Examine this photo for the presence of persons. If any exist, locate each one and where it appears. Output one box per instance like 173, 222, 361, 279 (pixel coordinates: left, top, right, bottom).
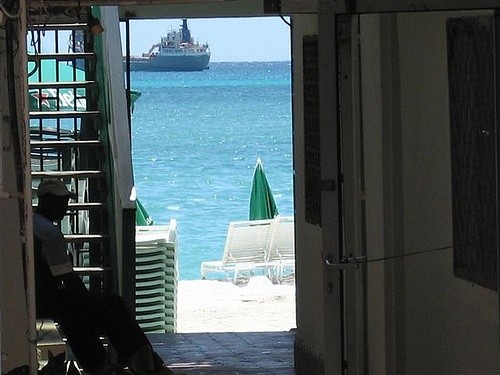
32, 179, 173, 375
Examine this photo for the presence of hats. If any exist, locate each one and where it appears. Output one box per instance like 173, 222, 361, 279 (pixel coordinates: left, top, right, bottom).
36, 177, 77, 202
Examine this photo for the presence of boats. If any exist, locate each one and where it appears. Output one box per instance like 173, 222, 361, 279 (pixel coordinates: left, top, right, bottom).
66, 18, 212, 74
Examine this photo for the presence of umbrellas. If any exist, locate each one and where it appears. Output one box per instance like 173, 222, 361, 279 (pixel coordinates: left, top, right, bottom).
135, 199, 153, 226
28, 51, 142, 115
249, 157, 279, 226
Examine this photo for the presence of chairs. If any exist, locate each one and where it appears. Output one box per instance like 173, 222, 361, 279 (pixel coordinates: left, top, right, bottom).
201, 216, 295, 286
